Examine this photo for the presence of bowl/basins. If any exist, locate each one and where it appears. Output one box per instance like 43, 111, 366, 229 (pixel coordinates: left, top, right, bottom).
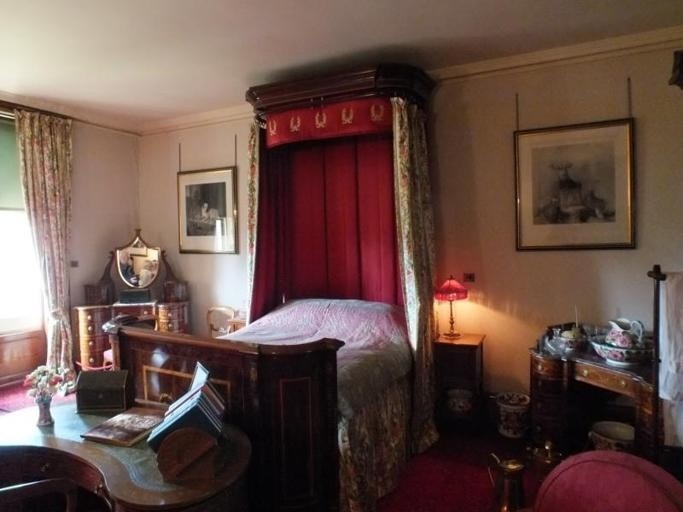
554, 334, 586, 350
589, 336, 653, 365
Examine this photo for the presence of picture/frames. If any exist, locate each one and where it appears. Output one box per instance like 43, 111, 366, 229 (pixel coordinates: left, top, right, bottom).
512, 118, 637, 252
175, 164, 238, 254
129, 238, 147, 257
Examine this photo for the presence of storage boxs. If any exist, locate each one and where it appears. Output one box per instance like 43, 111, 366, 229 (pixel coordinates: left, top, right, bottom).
78, 371, 135, 413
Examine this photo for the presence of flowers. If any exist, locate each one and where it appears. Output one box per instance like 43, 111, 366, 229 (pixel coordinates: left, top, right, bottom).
22, 365, 62, 403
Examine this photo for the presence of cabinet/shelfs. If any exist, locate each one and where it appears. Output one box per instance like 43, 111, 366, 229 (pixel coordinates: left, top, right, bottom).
70, 298, 191, 372
529, 347, 674, 474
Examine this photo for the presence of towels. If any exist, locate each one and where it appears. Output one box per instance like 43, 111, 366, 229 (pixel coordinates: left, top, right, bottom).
658, 271, 682, 446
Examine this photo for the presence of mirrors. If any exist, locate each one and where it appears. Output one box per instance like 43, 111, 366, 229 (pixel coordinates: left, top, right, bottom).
113, 228, 162, 289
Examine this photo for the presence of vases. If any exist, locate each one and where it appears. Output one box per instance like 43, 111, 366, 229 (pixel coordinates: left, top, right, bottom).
34, 397, 54, 428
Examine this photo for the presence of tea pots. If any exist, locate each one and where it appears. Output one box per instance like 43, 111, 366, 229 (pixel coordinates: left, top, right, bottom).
606, 319, 645, 349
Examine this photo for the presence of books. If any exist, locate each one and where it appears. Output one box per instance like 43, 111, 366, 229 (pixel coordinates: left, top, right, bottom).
147, 382, 227, 452
79, 407, 166, 448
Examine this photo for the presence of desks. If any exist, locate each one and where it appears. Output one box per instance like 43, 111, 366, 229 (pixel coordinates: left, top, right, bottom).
0, 397, 251, 511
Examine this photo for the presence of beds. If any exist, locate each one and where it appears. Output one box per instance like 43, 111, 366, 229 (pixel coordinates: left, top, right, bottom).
101, 296, 434, 512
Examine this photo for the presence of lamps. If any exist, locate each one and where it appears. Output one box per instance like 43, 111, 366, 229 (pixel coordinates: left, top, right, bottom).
434, 275, 466, 340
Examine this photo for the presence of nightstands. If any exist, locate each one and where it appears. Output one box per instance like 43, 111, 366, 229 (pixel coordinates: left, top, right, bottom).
227, 317, 246, 334
432, 332, 485, 421
